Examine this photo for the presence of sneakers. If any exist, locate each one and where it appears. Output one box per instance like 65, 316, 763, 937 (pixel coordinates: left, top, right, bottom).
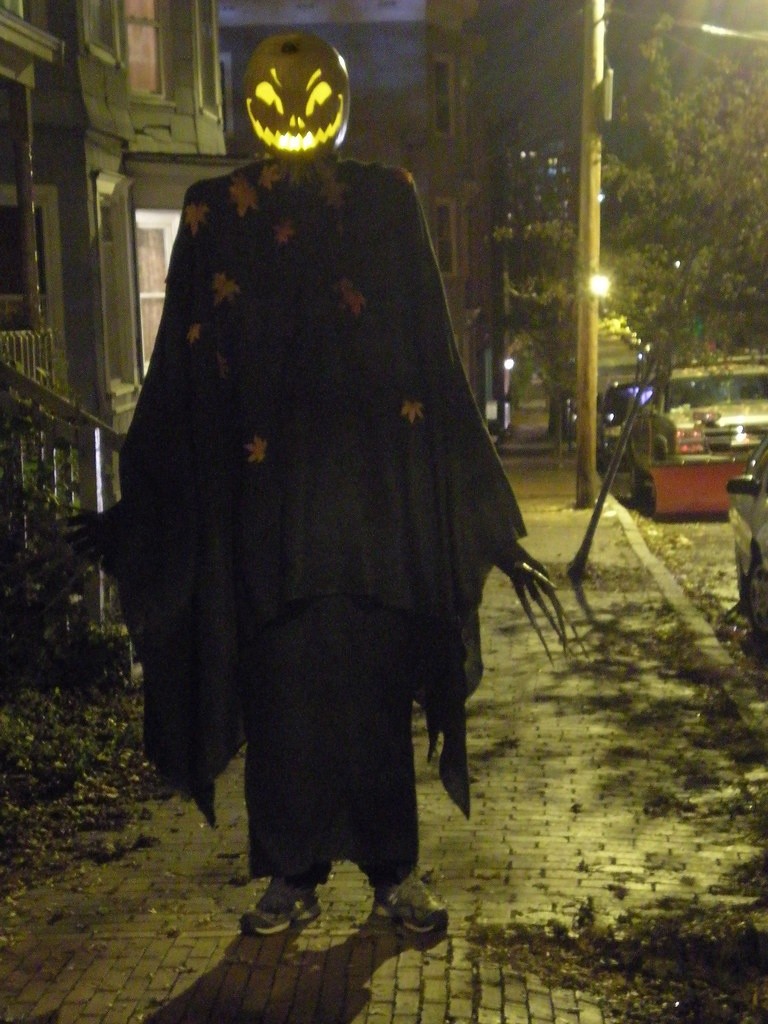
238, 880, 322, 935
373, 864, 448, 933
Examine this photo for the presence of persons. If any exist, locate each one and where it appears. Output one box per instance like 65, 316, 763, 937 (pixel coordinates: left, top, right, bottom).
11, 31, 590, 935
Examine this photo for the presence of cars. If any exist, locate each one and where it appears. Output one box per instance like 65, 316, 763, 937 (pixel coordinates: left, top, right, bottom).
725, 431, 768, 635
603, 375, 642, 470
630, 363, 768, 502
